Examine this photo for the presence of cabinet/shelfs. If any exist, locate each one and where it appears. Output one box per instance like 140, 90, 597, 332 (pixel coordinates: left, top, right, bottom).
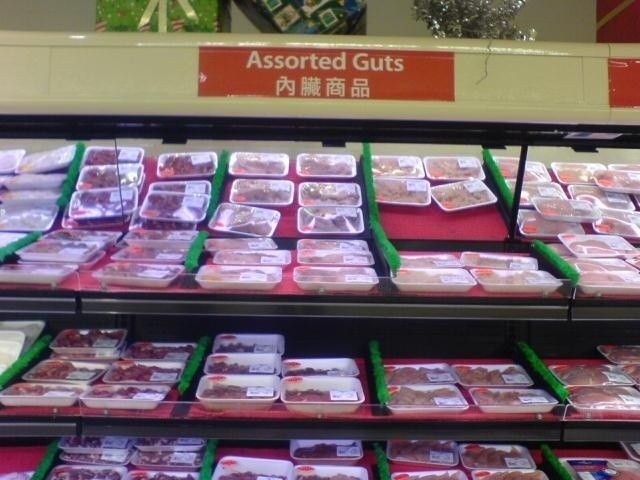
1, 113, 639, 479
